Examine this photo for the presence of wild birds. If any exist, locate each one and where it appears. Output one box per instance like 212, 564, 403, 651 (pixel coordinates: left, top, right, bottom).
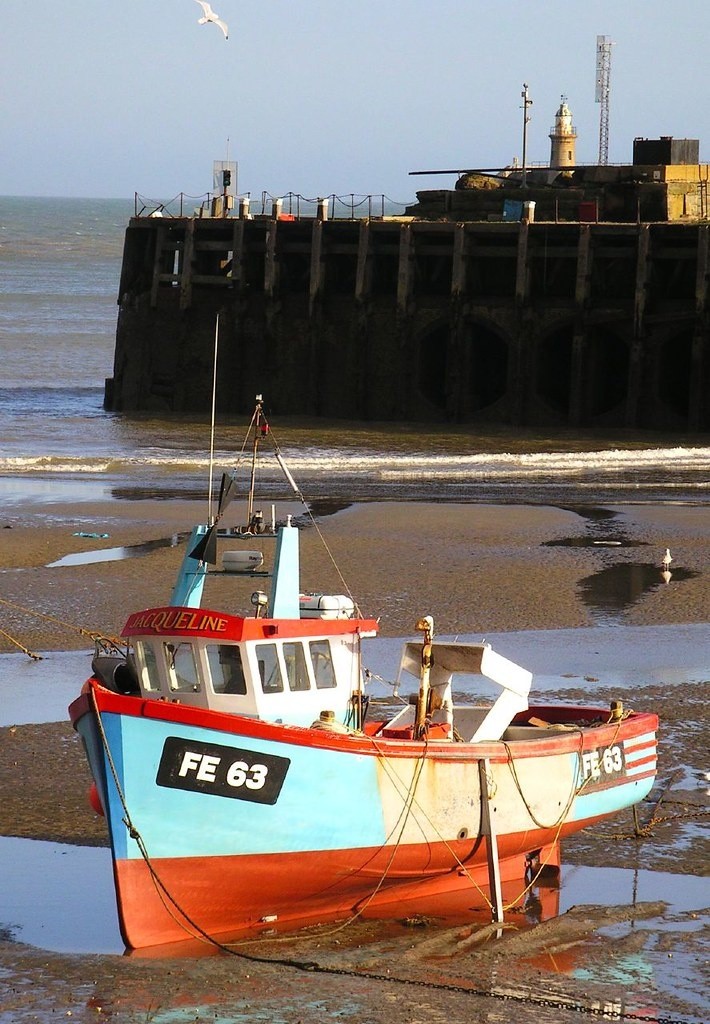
661, 548, 672, 567
660, 567, 672, 585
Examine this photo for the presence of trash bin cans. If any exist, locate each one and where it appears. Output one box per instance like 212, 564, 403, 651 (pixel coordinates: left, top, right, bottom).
523, 201, 537, 223
211, 196, 223, 218
239, 197, 250, 220
272, 198, 284, 221
317, 199, 329, 221
578, 201, 596, 223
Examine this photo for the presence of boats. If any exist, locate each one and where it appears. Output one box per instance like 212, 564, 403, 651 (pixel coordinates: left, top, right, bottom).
69, 394, 658, 959
